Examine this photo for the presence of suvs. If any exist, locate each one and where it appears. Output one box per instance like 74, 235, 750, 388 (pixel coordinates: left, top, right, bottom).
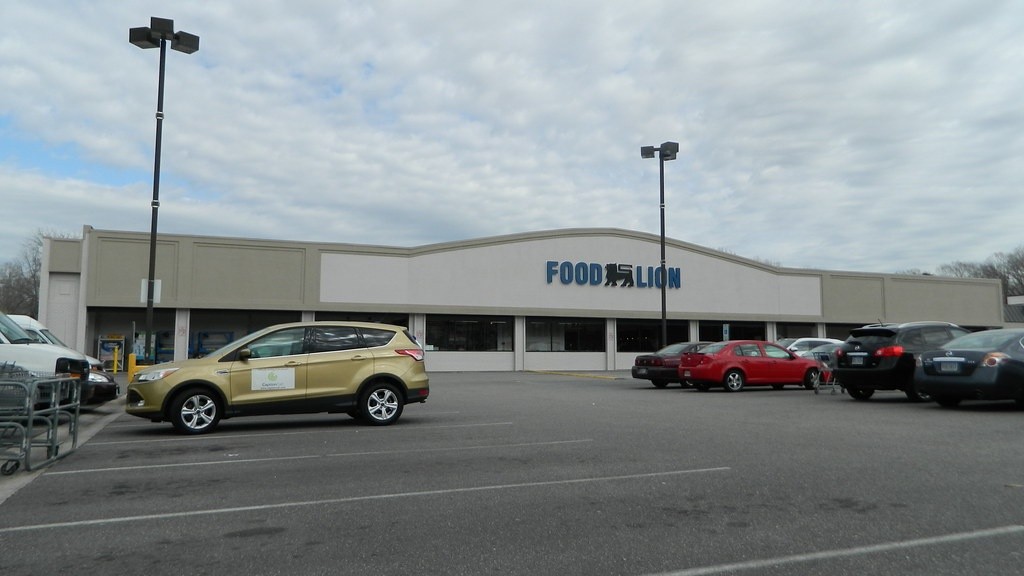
126, 320, 429, 435
830, 320, 974, 403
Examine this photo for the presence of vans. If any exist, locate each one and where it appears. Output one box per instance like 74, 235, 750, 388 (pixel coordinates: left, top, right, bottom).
0, 312, 90, 426
9, 315, 105, 375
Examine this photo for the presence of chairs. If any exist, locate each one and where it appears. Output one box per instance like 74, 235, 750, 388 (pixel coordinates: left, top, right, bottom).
290, 333, 305, 354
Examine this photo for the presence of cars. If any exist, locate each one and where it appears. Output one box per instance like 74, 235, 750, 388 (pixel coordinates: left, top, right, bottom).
752, 337, 845, 358
915, 327, 1024, 409
631, 341, 716, 388
679, 340, 832, 393
81, 368, 120, 411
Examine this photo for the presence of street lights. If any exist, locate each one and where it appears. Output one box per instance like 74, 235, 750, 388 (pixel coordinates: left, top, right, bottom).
641, 140, 680, 352
129, 17, 200, 368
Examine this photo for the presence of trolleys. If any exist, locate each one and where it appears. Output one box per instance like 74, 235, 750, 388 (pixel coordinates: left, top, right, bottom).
0, 364, 72, 476
811, 350, 847, 394
